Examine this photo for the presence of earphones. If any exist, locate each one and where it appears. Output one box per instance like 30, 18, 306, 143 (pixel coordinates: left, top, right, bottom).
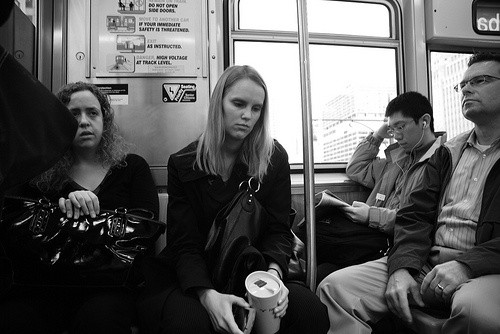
422, 121, 426, 127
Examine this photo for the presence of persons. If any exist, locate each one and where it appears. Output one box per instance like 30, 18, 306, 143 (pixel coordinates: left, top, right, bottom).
314, 46, 500, 334
296, 91, 448, 289
160, 64, 330, 334
24, 81, 158, 334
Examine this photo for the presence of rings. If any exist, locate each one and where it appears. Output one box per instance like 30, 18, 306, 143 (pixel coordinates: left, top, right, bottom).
438, 283, 444, 291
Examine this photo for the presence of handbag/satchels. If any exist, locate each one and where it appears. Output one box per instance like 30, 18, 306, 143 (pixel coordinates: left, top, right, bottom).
314, 209, 391, 266
1, 194, 167, 293
204, 176, 307, 283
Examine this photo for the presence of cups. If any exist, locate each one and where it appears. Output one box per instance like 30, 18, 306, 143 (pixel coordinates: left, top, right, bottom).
244, 271, 282, 334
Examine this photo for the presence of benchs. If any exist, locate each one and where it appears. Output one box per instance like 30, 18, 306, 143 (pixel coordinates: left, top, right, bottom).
409, 304, 450, 334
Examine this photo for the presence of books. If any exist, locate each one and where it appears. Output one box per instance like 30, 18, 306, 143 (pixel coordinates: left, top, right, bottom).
300, 188, 352, 228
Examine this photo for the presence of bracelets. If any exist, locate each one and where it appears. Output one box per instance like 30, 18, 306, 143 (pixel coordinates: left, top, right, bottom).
268, 268, 282, 280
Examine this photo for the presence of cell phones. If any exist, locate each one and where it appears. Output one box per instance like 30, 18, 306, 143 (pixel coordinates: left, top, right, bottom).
235, 305, 256, 334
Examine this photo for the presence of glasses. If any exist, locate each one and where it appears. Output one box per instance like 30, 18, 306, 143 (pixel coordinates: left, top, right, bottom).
453, 74, 500, 93
386, 119, 415, 138
